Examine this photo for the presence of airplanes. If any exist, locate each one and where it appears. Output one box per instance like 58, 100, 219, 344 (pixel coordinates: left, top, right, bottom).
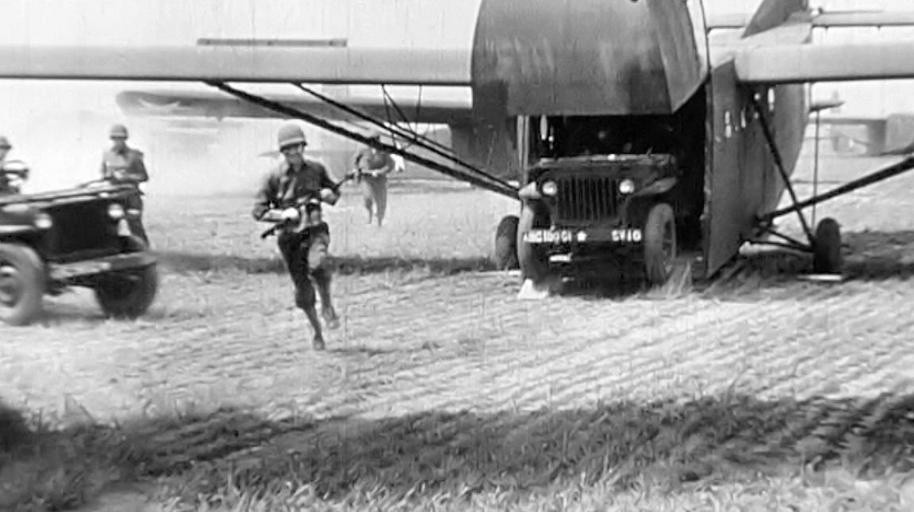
0, 0, 914, 288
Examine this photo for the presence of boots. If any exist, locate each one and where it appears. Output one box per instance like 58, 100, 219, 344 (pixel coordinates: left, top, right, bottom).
320, 293, 340, 329
307, 313, 325, 352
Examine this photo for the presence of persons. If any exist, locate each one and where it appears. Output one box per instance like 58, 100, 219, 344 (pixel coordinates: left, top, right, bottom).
353, 132, 394, 226
100, 125, 149, 247
0, 138, 28, 198
252, 125, 339, 351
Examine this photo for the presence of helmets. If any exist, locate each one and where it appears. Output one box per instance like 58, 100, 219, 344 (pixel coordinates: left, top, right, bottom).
0, 136, 11, 148
367, 129, 379, 139
277, 124, 306, 152
109, 123, 128, 140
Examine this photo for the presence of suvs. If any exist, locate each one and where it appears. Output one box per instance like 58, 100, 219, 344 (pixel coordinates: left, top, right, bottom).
513, 144, 684, 287
0, 159, 162, 327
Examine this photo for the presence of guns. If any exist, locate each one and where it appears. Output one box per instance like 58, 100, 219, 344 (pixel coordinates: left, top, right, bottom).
261, 173, 356, 238
